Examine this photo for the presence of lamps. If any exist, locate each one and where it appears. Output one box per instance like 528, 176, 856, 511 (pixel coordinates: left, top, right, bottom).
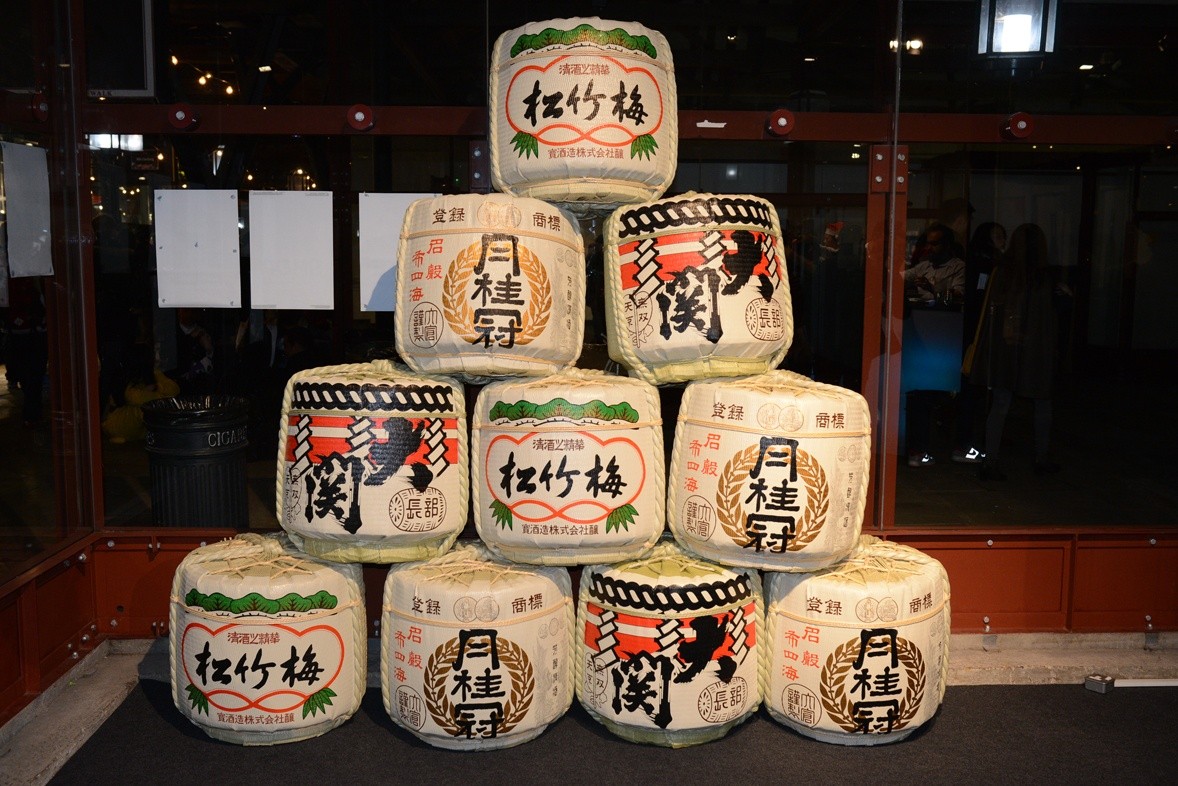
968, 0, 1063, 120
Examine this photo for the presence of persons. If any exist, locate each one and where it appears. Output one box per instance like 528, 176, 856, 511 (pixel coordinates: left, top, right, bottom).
0, 115, 1013, 503
974, 223, 1063, 482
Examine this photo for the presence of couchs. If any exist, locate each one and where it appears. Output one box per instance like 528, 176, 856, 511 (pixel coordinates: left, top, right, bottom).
841, 299, 964, 396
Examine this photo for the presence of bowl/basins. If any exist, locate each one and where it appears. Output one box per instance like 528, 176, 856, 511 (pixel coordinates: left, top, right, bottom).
923, 299, 936, 307
